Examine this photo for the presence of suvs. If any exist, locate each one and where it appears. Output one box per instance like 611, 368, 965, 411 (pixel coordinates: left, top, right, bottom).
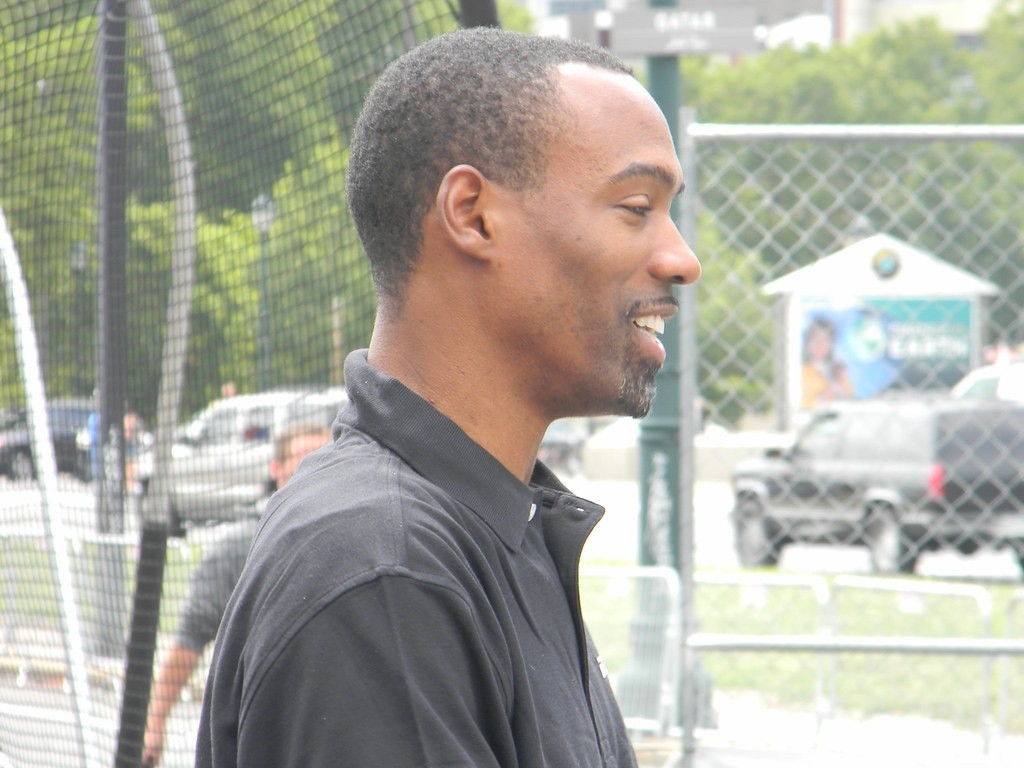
727, 391, 1024, 574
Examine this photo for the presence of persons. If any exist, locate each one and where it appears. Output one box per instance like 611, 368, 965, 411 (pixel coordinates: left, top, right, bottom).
86, 389, 145, 517
801, 319, 854, 409
140, 421, 334, 767
194, 26, 699, 768
981, 339, 1024, 367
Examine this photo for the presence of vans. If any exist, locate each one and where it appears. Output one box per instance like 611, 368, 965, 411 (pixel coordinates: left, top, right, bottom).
135, 393, 345, 535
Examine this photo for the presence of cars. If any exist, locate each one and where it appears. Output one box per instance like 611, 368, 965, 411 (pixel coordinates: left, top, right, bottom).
0, 400, 105, 481
